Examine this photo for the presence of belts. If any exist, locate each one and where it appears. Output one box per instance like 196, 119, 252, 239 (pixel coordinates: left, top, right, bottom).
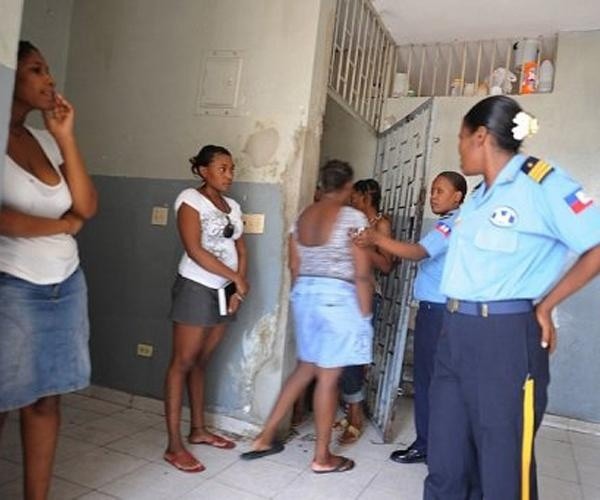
445, 299, 532, 317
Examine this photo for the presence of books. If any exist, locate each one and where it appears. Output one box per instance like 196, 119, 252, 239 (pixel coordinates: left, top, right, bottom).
218, 282, 237, 316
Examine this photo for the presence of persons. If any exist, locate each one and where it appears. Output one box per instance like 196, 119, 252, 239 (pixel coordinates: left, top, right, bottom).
160, 144, 252, 474
294, 184, 324, 429
346, 169, 468, 465
0, 39, 101, 500
421, 95, 600, 499
238, 157, 377, 474
332, 179, 396, 446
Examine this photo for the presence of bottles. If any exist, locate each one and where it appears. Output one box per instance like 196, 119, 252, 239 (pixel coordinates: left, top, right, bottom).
450, 78, 502, 95
540, 59, 554, 94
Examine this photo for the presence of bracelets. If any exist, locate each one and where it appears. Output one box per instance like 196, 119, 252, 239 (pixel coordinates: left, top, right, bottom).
234, 292, 244, 301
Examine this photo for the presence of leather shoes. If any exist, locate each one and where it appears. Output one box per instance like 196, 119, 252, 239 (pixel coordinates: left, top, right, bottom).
390, 446, 427, 464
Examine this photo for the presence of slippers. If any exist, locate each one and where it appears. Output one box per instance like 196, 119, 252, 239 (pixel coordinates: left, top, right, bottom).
190, 433, 236, 449
242, 444, 284, 459
164, 451, 206, 472
314, 455, 355, 473
332, 417, 350, 431
335, 423, 363, 447
